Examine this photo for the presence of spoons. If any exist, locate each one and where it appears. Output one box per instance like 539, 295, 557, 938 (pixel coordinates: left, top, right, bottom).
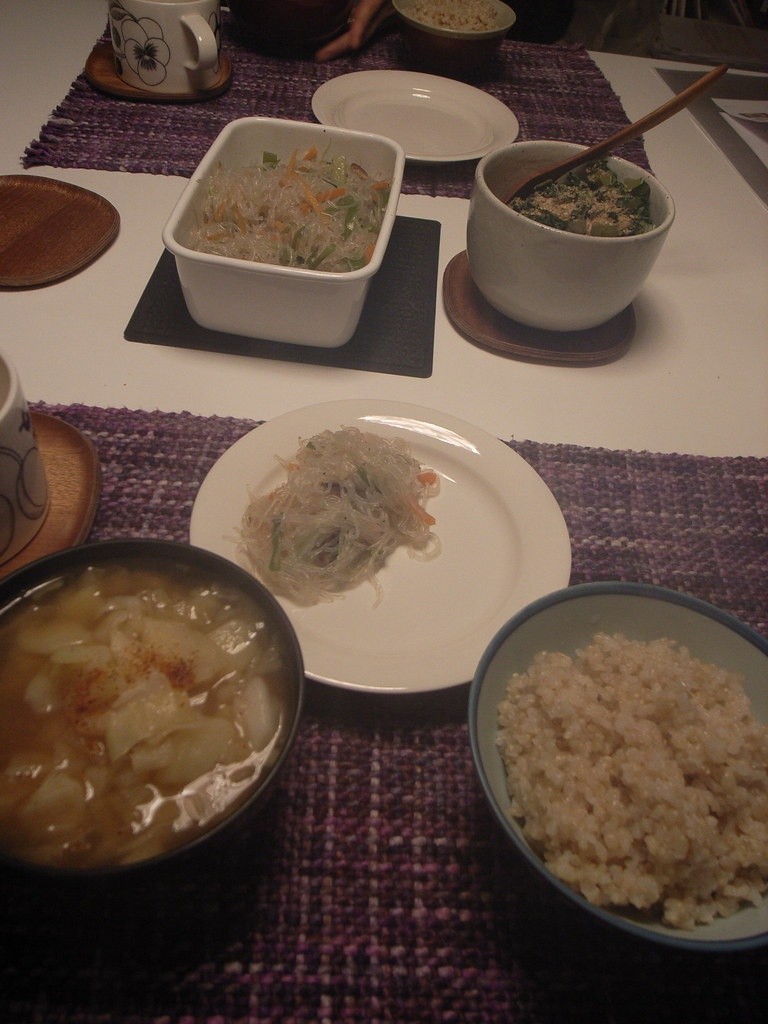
501, 64, 729, 205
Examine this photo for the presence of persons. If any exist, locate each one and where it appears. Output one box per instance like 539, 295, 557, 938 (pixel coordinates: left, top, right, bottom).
315, 0, 577, 63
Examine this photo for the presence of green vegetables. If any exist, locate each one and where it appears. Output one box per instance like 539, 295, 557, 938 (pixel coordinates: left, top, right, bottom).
235, 157, 390, 271
511, 159, 653, 238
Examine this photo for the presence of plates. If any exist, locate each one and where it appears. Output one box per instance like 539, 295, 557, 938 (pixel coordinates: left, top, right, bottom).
311, 70, 520, 165
189, 398, 571, 694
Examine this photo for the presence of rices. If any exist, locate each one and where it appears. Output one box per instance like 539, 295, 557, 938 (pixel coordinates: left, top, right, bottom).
400, 0, 498, 31
492, 632, 768, 930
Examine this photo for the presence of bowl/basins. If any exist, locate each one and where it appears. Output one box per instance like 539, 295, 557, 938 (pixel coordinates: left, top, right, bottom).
466, 140, 675, 331
0, 538, 305, 879
391, 0, 517, 72
469, 581, 768, 950
161, 116, 405, 349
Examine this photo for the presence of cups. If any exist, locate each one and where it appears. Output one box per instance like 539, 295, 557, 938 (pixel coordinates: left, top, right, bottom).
108, 0, 222, 94
0, 357, 50, 565
